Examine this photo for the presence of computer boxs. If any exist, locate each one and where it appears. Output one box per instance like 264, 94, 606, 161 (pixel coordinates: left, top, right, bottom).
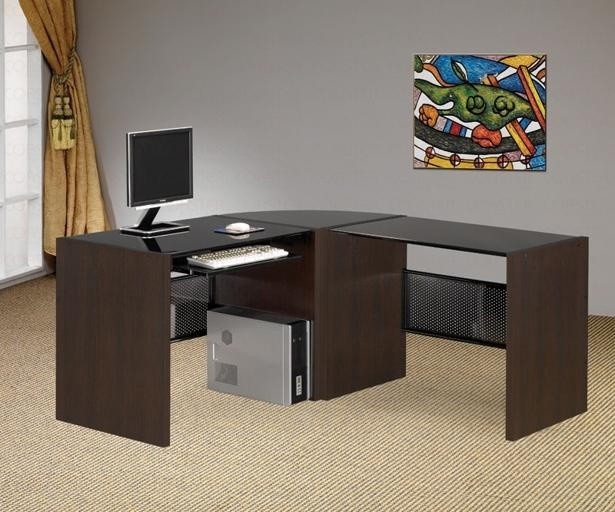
206, 309, 313, 406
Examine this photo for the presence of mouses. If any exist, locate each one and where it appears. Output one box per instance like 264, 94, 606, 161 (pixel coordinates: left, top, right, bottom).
225, 221, 251, 233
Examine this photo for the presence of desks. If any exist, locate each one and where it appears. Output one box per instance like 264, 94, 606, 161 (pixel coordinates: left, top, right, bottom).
54, 210, 590, 448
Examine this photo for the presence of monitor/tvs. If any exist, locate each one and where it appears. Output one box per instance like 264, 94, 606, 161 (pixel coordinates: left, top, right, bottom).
119, 126, 194, 237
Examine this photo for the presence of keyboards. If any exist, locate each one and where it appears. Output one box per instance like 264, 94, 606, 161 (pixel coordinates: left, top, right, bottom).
187, 243, 289, 271
